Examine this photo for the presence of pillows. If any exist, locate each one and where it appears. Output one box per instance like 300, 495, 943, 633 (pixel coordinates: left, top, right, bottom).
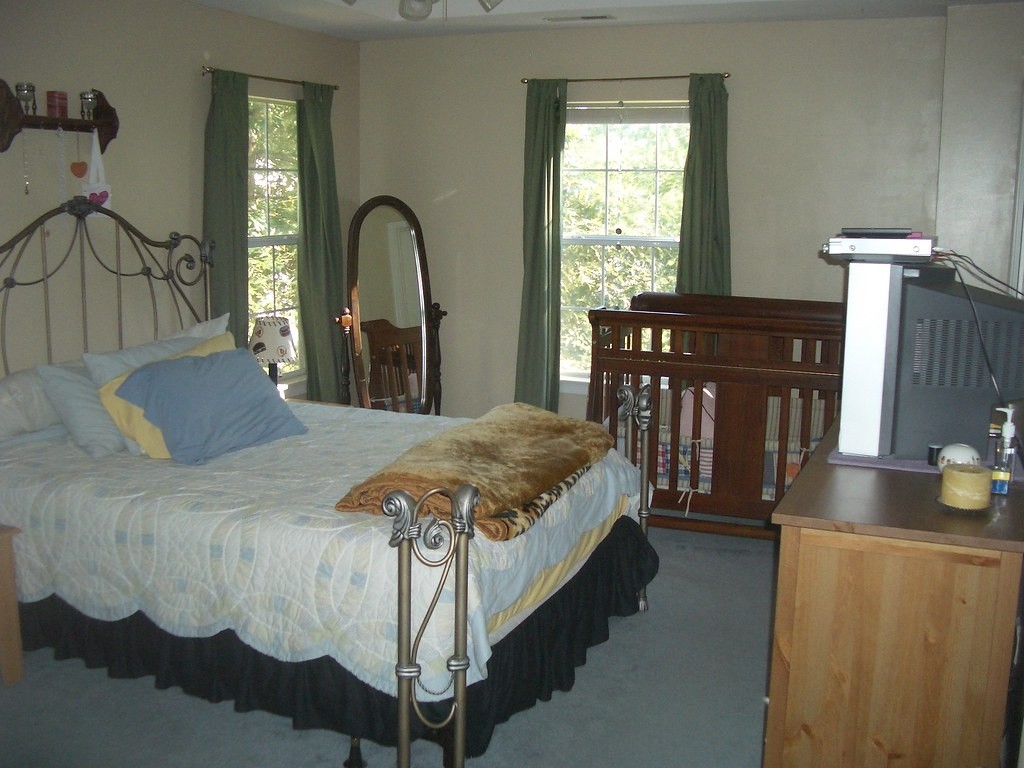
38, 311, 308, 468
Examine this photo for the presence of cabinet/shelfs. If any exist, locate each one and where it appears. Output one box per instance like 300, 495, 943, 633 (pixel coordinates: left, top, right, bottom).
761, 404, 1024, 768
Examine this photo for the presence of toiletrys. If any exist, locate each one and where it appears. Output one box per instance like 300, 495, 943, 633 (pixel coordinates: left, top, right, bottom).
991, 407, 1016, 495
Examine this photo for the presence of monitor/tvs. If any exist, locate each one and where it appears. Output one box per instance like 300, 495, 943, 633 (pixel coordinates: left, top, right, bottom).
838, 262, 1024, 463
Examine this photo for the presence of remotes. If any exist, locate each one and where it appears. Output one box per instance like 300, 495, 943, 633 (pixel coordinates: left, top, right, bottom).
842, 227, 912, 239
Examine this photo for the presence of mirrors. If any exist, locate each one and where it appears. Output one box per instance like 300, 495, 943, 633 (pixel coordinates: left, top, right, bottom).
330, 195, 447, 416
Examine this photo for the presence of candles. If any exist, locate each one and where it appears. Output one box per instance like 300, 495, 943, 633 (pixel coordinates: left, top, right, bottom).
47, 90, 68, 118
941, 457, 992, 510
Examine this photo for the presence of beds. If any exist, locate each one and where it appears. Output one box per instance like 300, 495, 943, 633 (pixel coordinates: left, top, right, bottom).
0, 194, 659, 768
585, 290, 847, 544
360, 320, 422, 415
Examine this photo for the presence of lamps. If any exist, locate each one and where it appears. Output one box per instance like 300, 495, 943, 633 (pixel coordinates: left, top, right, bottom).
0, 77, 119, 196
247, 316, 298, 388
341, 0, 504, 24
